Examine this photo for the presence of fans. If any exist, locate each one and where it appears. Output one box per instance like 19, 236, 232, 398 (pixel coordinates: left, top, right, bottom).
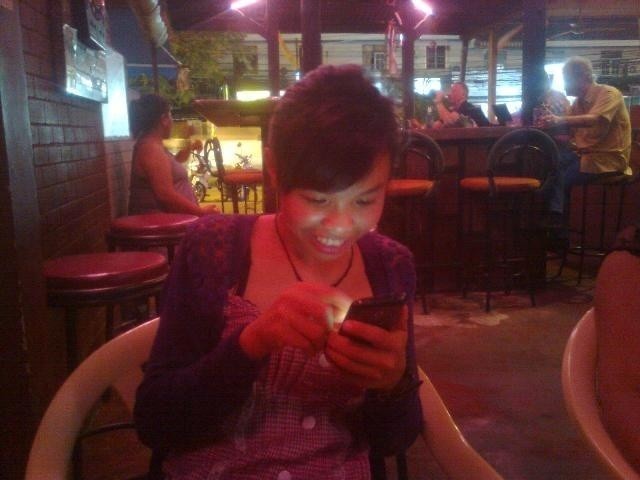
545, 9, 626, 41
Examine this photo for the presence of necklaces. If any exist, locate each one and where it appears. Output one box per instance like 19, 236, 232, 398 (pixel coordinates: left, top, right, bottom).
273, 210, 356, 289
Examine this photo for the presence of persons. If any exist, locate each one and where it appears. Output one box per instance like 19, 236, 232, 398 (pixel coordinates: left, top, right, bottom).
132, 65, 424, 480
530, 55, 632, 253
502, 70, 573, 128
126, 93, 222, 261
430, 82, 490, 130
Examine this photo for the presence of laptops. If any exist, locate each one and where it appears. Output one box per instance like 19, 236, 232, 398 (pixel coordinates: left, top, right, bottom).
467, 107, 504, 127
493, 104, 513, 126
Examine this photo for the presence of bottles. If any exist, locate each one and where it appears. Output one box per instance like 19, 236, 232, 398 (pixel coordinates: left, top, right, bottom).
223, 77, 229, 101
425, 105, 433, 130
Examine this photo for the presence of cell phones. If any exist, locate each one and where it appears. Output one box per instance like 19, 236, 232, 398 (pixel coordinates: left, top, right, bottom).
338, 294, 402, 341
